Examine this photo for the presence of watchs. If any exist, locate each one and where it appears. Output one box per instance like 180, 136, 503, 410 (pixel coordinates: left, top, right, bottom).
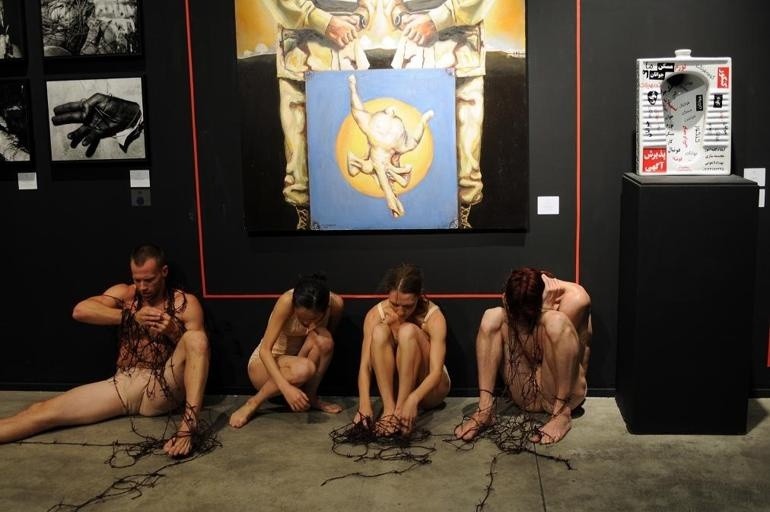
393, 11, 408, 29
353, 11, 367, 29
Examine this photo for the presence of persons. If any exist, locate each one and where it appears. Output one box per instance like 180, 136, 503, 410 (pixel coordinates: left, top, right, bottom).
352, 263, 451, 437
384, 0, 496, 229
454, 265, 593, 445
259, 0, 379, 230
0, 243, 211, 458
229, 269, 341, 429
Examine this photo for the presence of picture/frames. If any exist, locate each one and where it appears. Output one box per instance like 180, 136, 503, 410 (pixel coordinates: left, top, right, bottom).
0, 0, 29, 66
0, 77, 36, 167
43, 74, 148, 164
38, 0, 145, 62
